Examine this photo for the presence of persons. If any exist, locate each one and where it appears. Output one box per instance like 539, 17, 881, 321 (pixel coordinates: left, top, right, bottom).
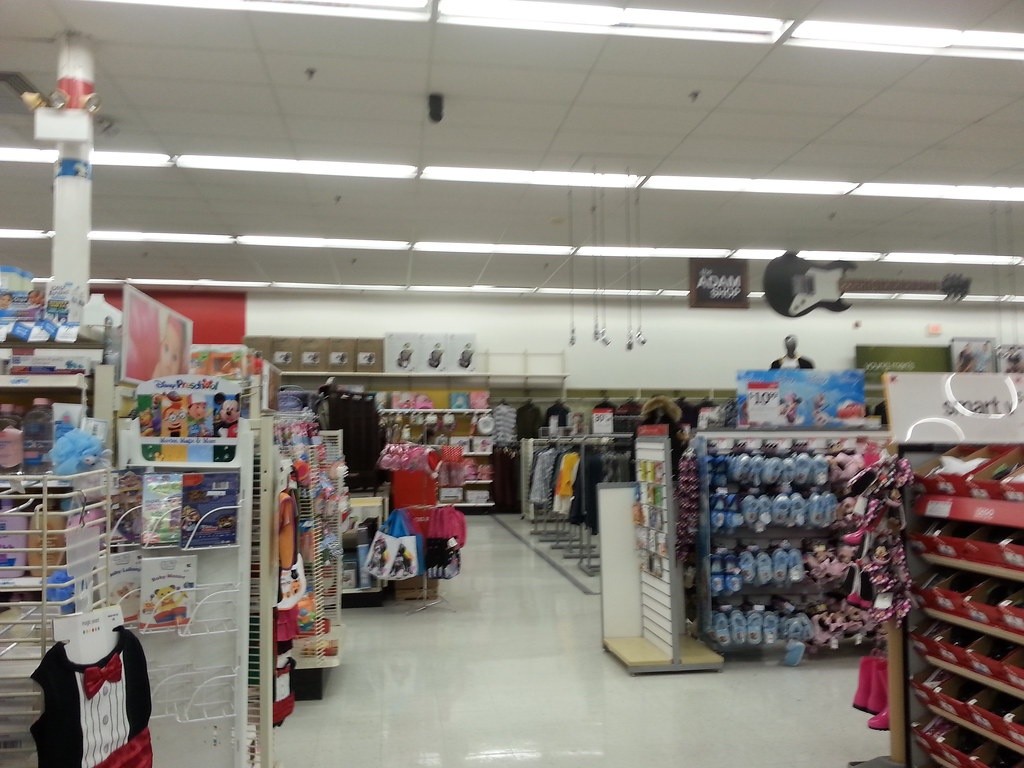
771, 335, 814, 370
153, 314, 186, 380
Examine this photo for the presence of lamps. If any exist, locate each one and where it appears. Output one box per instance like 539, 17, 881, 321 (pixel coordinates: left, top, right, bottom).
19, 87, 119, 143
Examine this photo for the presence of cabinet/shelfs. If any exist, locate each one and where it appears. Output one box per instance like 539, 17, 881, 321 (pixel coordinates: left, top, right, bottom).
517, 368, 1023, 768
0, 282, 493, 768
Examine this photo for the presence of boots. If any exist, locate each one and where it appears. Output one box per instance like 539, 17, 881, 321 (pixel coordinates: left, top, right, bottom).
868, 665, 890, 731
853, 655, 887, 714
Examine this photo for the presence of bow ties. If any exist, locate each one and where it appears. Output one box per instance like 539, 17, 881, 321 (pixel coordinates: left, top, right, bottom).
84, 653, 122, 701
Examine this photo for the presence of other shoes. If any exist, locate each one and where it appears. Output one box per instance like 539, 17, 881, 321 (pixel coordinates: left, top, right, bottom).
847, 593, 872, 610
709, 548, 804, 597
842, 524, 863, 546
784, 642, 805, 666
707, 454, 829, 488
711, 609, 813, 646
710, 492, 838, 531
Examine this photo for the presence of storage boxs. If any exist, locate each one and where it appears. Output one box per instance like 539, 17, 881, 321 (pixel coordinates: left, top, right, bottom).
465, 490, 490, 502
440, 488, 462, 502
